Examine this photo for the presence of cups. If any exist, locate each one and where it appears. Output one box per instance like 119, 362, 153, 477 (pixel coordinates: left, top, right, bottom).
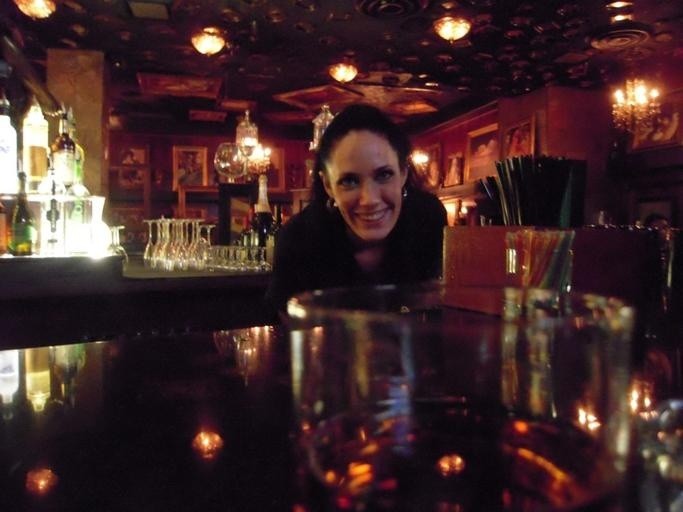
143, 219, 156, 270
204, 223, 216, 273
212, 142, 249, 183
174, 219, 189, 271
256, 246, 274, 272
190, 219, 205, 273
247, 246, 261, 272
217, 245, 226, 273
233, 245, 246, 272
157, 219, 173, 270
227, 245, 236, 272
286, 282, 639, 512
152, 218, 164, 270
105, 225, 129, 272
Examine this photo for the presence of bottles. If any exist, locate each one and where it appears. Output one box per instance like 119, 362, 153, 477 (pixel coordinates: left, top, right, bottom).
636, 221, 641, 225
454, 200, 468, 226
22, 106, 48, 194
8, 172, 37, 256
0, 115, 20, 194
66, 128, 84, 183
236, 204, 258, 247
659, 228, 683, 339
52, 111, 75, 193
251, 175, 273, 248
268, 201, 283, 266
598, 211, 608, 226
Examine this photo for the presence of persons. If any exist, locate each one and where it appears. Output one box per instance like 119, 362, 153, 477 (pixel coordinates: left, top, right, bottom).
272, 103, 448, 311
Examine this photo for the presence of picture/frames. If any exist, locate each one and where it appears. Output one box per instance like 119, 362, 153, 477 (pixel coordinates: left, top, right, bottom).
118, 144, 151, 189
189, 109, 226, 122
466, 122, 498, 179
268, 148, 285, 193
389, 98, 438, 116
498, 111, 537, 161
138, 73, 222, 100
274, 83, 364, 113
219, 183, 254, 246
442, 153, 464, 187
172, 146, 208, 192
217, 97, 256, 115
